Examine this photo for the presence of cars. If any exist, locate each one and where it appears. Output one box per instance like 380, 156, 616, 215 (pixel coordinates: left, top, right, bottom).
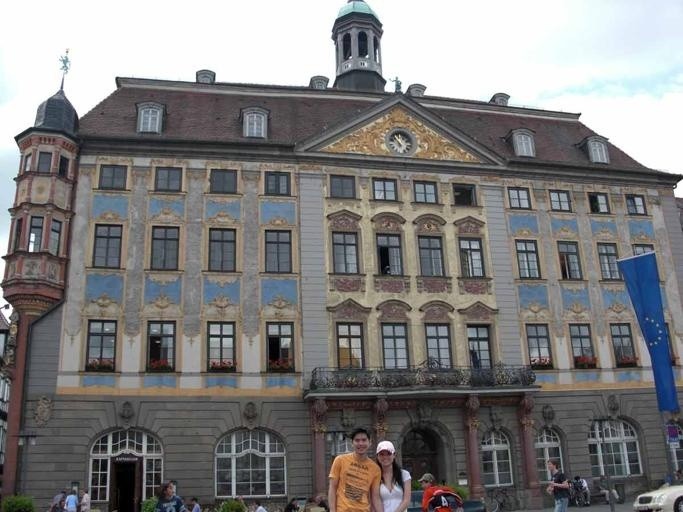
632, 476, 682, 512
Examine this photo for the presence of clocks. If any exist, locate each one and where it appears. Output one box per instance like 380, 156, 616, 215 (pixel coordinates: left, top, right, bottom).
386, 128, 416, 155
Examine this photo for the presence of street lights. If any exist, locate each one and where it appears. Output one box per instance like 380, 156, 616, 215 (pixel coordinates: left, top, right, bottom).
586, 415, 616, 511
11, 430, 43, 498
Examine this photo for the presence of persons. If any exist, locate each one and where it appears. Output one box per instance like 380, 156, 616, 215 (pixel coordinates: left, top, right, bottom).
597, 475, 620, 504
156, 482, 201, 512
50, 490, 91, 512
285, 428, 412, 512
418, 473, 454, 512
547, 459, 591, 512
251, 500, 267, 512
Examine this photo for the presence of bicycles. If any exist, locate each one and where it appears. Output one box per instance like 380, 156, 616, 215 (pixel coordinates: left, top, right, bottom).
567, 480, 586, 507
484, 486, 517, 511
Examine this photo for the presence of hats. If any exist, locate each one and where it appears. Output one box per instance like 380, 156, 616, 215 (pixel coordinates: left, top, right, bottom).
418, 474, 434, 483
377, 441, 395, 455
